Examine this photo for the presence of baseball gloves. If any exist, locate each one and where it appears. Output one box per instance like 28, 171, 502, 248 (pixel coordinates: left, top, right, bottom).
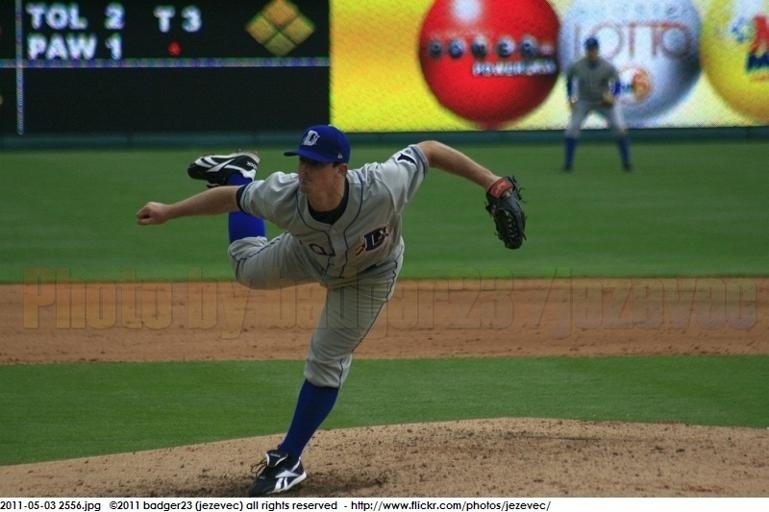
485, 176, 527, 249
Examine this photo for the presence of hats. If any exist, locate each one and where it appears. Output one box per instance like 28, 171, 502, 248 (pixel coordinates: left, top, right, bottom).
583, 41, 599, 51
284, 125, 350, 163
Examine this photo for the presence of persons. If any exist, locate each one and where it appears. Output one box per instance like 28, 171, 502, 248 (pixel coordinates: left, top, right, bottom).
136, 125, 524, 496
563, 37, 632, 172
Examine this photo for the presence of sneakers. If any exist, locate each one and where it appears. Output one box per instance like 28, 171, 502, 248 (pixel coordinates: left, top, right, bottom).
624, 163, 631, 172
188, 152, 260, 187
559, 163, 572, 173
247, 451, 306, 497
